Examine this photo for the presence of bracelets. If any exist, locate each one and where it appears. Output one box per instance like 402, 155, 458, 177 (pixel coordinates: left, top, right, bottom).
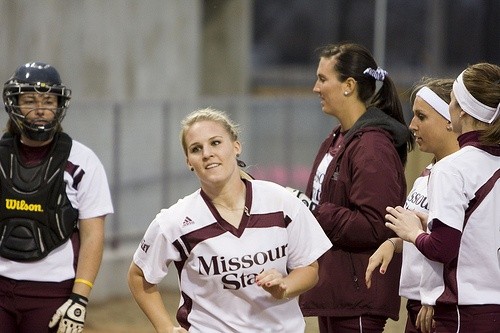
75, 279, 94, 288
388, 238, 397, 249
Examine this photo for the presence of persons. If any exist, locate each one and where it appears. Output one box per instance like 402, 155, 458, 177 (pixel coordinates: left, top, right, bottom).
128, 109, 332, 333
0, 62, 114, 333
366, 79, 461, 333
385, 62, 500, 333
285, 44, 417, 333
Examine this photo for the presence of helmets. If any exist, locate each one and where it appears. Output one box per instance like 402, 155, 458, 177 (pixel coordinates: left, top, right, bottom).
2, 61, 72, 142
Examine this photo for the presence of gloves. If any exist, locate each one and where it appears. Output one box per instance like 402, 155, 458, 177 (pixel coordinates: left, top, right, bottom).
48, 291, 89, 333
285, 186, 318, 212
